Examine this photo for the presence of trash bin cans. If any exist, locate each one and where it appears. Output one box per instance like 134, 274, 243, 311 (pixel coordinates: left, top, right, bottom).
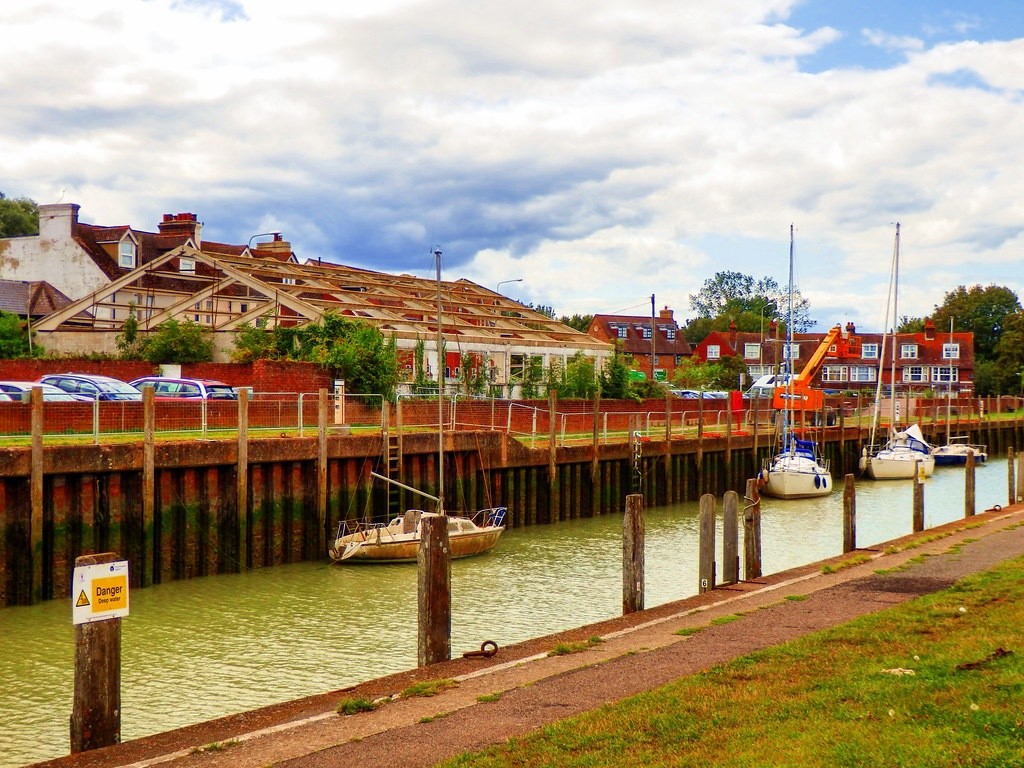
843, 402, 852, 417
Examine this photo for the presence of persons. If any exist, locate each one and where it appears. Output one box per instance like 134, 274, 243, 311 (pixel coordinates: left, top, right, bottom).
846, 322, 856, 336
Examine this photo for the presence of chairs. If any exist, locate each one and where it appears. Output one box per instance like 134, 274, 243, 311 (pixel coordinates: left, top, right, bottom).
160, 386, 169, 392
178, 386, 188, 392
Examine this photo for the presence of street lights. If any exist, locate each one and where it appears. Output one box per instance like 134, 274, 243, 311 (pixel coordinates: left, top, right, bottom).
496, 278, 523, 293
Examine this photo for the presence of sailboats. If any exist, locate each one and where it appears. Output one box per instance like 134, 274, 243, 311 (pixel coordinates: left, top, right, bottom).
931, 315, 988, 467
329, 242, 508, 566
755, 221, 836, 502
857, 221, 937, 481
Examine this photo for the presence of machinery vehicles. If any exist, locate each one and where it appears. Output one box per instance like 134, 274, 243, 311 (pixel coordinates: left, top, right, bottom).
772, 325, 862, 425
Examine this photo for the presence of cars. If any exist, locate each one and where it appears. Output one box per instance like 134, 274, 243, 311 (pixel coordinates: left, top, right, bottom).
672, 389, 772, 399
0, 371, 145, 402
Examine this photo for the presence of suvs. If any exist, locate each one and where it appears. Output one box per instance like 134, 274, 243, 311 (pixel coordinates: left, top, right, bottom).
127, 376, 238, 400
